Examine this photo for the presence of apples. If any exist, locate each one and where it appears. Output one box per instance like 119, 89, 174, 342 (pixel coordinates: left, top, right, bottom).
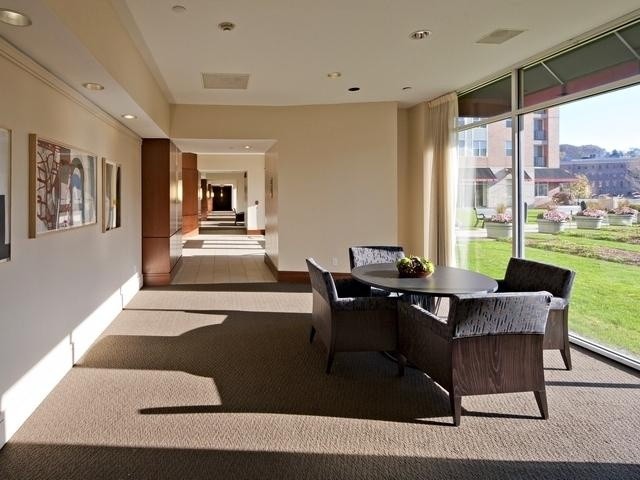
398, 256, 434, 271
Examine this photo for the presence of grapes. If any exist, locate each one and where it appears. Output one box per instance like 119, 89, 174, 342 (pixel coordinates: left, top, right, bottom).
396, 260, 424, 275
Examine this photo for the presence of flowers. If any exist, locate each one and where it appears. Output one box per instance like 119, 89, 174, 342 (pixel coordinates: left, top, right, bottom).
608, 205, 638, 216
576, 208, 606, 218
484, 211, 512, 223
537, 209, 572, 222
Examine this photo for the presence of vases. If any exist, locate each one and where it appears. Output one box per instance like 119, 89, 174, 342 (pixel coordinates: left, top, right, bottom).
607, 215, 634, 227
574, 216, 603, 229
484, 222, 512, 238
536, 219, 562, 233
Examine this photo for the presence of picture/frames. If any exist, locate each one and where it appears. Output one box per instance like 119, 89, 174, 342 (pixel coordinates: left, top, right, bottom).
1, 127, 13, 264
29, 133, 98, 239
102, 157, 122, 233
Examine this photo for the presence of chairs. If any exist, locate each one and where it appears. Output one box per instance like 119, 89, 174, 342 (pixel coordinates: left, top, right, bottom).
232, 207, 245, 225
348, 245, 435, 313
398, 290, 554, 426
305, 258, 397, 373
496, 257, 576, 370
474, 208, 484, 227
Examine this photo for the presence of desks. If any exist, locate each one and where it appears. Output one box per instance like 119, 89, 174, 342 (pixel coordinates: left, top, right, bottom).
351, 262, 499, 371
